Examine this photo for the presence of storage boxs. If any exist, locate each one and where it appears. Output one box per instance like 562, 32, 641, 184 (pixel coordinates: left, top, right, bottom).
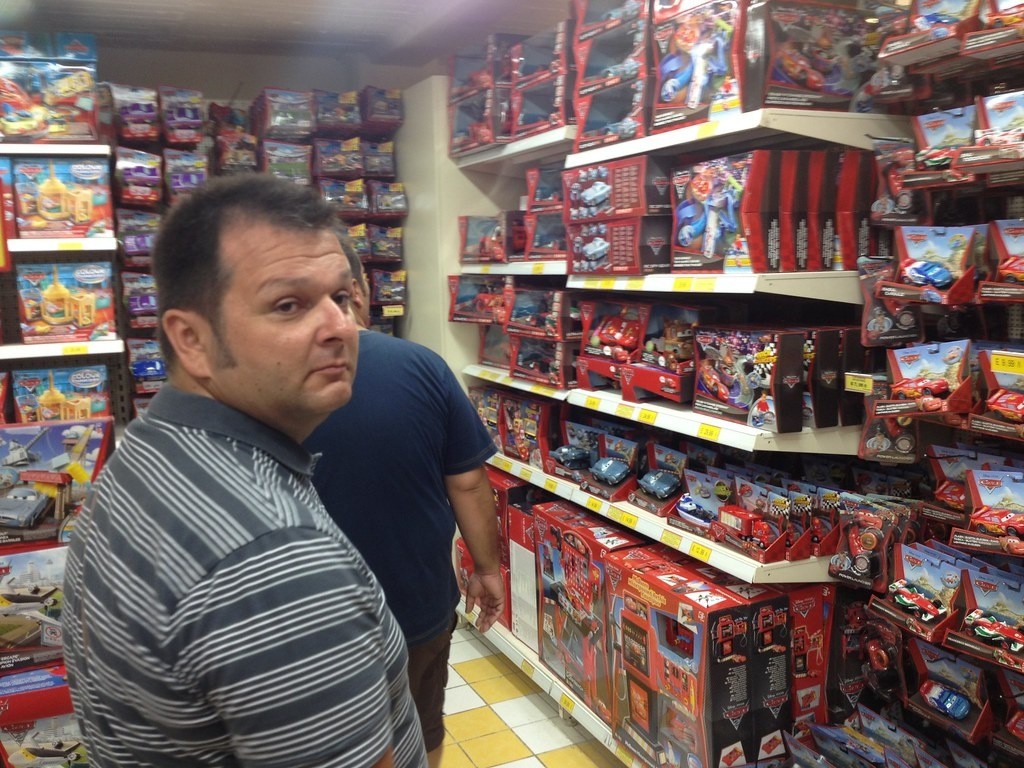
620, 363, 694, 406
453, 463, 837, 768
565, 214, 674, 274
652, 0, 877, 134
690, 325, 804, 434
0, 541, 92, 768
478, 322, 516, 368
502, 285, 585, 339
561, 154, 678, 225
574, 356, 624, 392
450, 0, 650, 156
838, 323, 862, 427
459, 166, 562, 263
0, 32, 410, 539
670, 147, 888, 272
579, 297, 647, 362
448, 276, 518, 325
784, 328, 838, 428
635, 299, 723, 375
507, 330, 579, 389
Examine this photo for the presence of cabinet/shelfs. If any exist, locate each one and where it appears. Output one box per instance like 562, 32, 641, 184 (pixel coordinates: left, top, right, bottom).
0, 63, 129, 428
450, 0, 1024, 768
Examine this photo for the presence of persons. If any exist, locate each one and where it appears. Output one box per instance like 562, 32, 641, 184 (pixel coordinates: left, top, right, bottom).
55, 172, 433, 768
293, 230, 505, 768
